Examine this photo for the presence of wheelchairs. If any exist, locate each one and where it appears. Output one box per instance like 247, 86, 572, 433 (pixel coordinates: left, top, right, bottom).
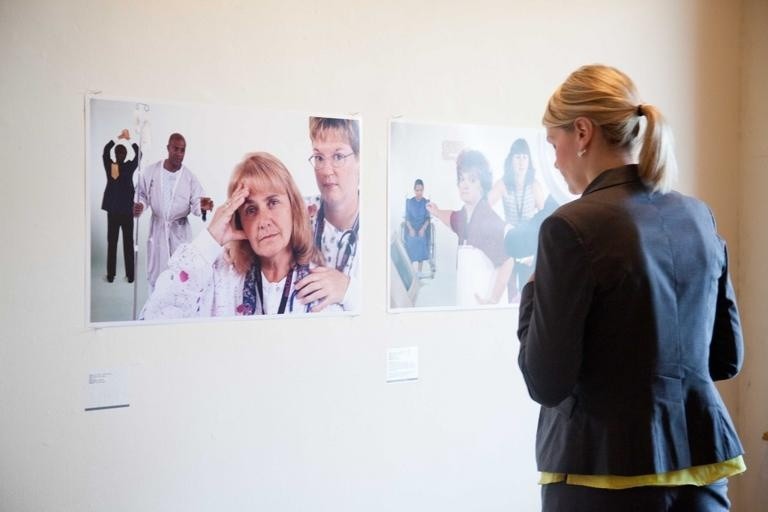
401, 198, 436, 280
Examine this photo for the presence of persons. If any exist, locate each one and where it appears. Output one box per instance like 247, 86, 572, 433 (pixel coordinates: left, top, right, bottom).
101, 128, 143, 283
138, 153, 347, 321
133, 133, 213, 296
516, 64, 748, 512
405, 139, 544, 306
223, 116, 359, 312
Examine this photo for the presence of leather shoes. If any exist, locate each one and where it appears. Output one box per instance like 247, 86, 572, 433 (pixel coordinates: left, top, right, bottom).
108, 275, 114, 282
128, 277, 134, 283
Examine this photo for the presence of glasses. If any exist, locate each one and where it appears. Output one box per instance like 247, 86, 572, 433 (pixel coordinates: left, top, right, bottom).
309, 152, 354, 169
